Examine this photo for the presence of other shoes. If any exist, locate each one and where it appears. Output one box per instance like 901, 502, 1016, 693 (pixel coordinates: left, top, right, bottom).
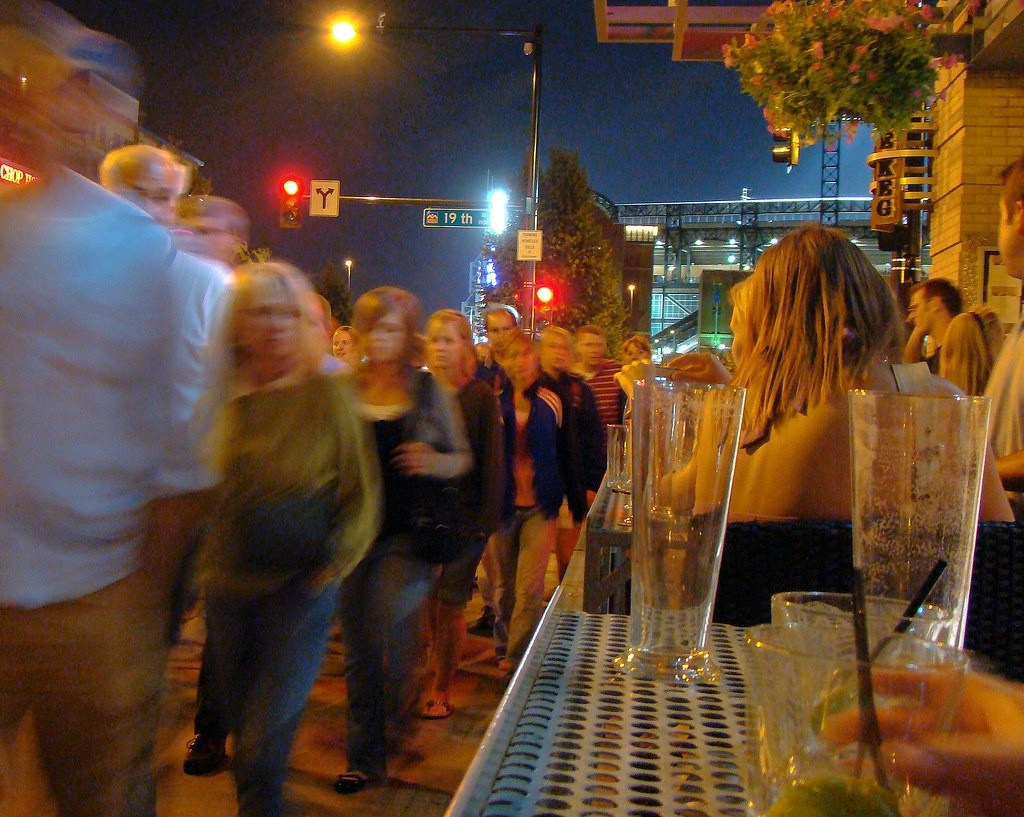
497, 669, 516, 694
335, 769, 387, 794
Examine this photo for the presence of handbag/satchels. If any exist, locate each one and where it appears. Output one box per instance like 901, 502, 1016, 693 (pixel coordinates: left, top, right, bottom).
414, 487, 471, 564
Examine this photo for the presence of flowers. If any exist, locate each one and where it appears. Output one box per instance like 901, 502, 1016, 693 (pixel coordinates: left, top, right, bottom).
721, 0, 959, 139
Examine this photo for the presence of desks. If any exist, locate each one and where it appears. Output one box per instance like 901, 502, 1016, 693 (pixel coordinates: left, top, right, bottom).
584, 464, 635, 617
443, 587, 761, 816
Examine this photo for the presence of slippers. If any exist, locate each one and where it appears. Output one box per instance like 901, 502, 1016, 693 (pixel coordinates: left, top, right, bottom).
422, 698, 454, 717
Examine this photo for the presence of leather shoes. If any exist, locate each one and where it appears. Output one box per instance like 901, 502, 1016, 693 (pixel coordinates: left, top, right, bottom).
467, 607, 495, 636
183, 732, 225, 774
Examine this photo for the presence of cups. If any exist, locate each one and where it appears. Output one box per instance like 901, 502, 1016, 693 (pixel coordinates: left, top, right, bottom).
606, 424, 653, 495
847, 387, 996, 650
744, 623, 969, 817
611, 370, 746, 684
771, 591, 957, 641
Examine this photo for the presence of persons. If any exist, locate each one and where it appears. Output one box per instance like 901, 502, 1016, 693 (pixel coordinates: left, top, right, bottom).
976, 155, 1024, 496
570, 323, 625, 437
901, 278, 964, 373
616, 226, 1013, 521
821, 666, 1024, 817
939, 303, 1005, 396
0, 139, 605, 817
619, 336, 653, 424
